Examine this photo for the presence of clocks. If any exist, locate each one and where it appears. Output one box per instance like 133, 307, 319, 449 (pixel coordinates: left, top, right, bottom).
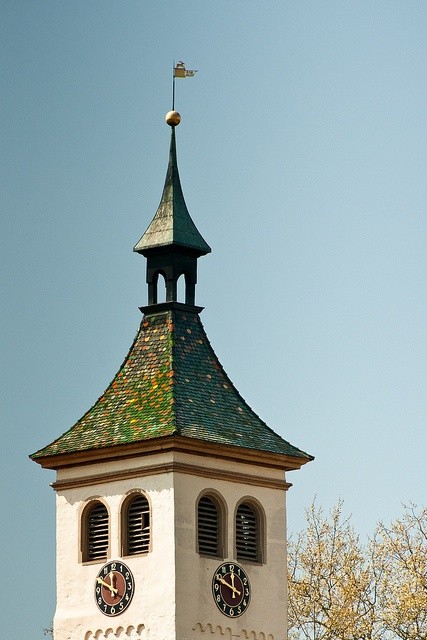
212, 561, 252, 619
93, 560, 134, 617
